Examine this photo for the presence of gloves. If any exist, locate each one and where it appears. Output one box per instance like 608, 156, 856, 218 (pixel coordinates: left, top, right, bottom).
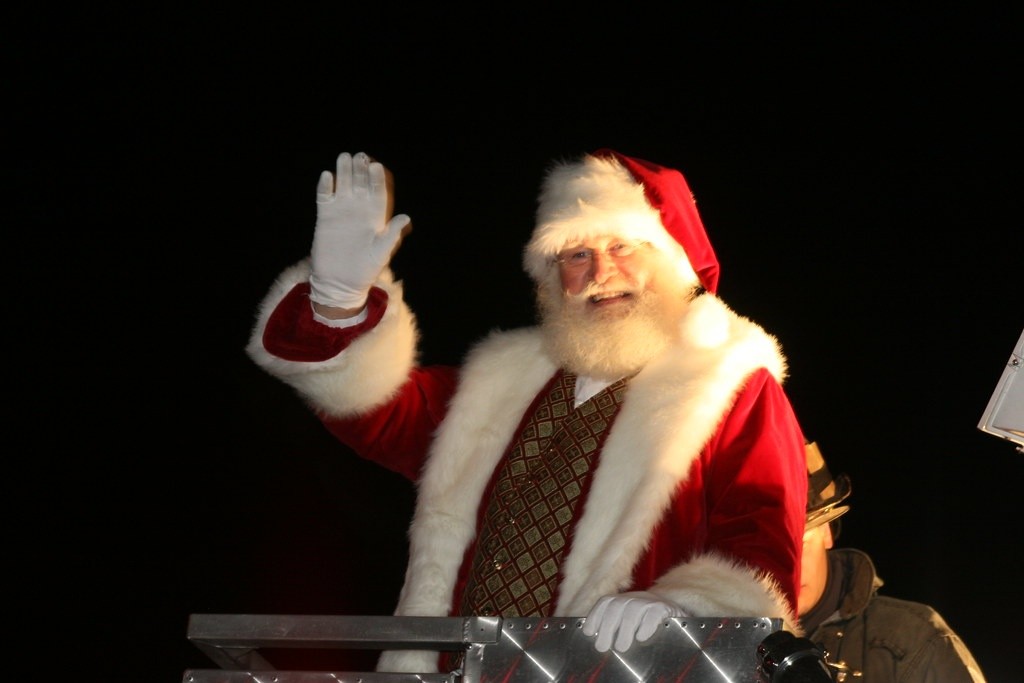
581, 589, 689, 653
308, 152, 412, 311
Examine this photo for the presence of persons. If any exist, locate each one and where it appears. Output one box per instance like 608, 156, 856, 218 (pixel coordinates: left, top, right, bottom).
795, 441, 987, 683
243, 146, 808, 683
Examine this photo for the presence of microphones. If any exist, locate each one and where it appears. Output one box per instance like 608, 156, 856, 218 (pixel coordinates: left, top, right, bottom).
756, 629, 836, 683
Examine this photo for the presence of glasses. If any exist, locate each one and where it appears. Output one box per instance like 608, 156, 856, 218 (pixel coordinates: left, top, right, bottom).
549, 242, 634, 266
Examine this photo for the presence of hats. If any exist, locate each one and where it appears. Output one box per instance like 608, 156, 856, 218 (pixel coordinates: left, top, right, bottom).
520, 148, 721, 296
805, 441, 853, 531
